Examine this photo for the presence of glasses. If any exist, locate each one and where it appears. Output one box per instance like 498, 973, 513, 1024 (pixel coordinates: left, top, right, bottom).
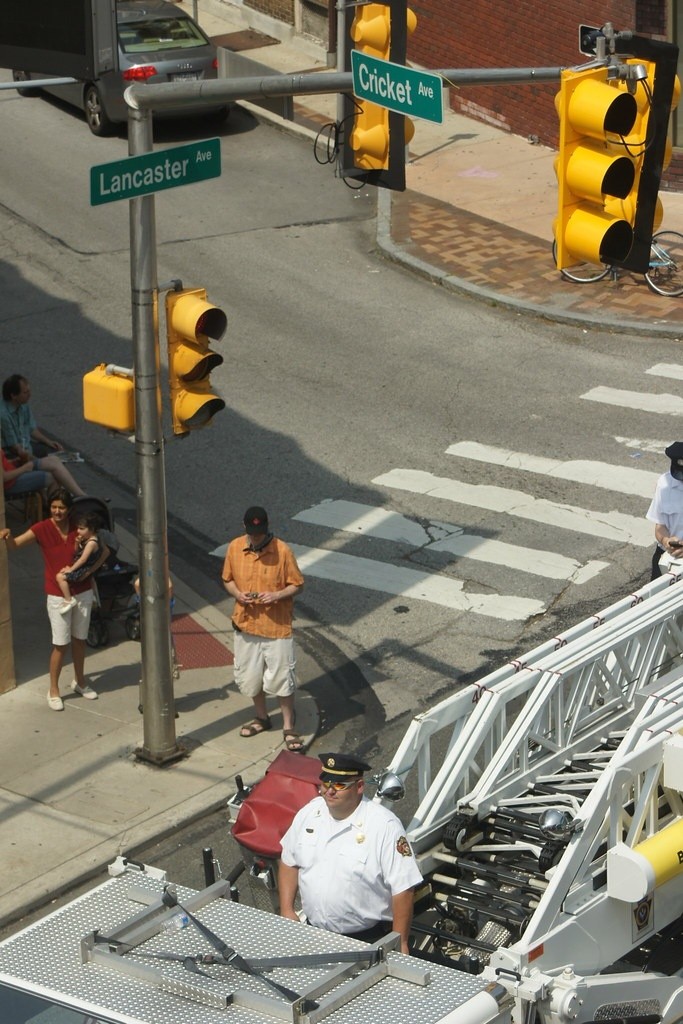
322, 780, 355, 790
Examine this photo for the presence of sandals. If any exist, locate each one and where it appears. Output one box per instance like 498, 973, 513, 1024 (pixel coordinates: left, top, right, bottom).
283, 730, 303, 751
240, 714, 271, 737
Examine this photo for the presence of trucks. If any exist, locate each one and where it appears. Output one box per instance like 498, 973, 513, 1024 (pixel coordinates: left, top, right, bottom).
0, 534, 683, 1024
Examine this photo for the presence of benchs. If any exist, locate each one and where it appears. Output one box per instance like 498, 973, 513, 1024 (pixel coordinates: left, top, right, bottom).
119, 28, 189, 44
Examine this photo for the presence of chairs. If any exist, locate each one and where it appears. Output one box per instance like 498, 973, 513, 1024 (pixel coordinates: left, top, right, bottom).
5, 489, 43, 544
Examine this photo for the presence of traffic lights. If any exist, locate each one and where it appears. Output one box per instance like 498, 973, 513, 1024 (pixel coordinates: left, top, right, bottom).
625, 59, 682, 238
555, 65, 636, 270
156, 283, 227, 437
350, 2, 418, 170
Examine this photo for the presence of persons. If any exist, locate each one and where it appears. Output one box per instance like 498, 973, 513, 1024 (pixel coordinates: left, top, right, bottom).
0, 490, 110, 710
646, 441, 683, 581
279, 753, 423, 954
222, 507, 305, 751
56, 512, 103, 614
0, 374, 111, 503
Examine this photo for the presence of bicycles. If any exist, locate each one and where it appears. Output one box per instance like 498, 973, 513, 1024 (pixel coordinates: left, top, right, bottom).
552, 230, 683, 297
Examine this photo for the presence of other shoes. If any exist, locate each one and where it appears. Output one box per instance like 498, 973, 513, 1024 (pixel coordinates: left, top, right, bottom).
71, 680, 98, 699
60, 597, 77, 615
93, 597, 98, 607
47, 690, 63, 710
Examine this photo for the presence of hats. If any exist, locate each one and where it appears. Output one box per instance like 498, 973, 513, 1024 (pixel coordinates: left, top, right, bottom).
243, 506, 268, 534
318, 753, 372, 782
666, 441, 683, 481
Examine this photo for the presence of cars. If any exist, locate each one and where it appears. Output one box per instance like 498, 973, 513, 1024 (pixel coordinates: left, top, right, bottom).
10, 0, 233, 138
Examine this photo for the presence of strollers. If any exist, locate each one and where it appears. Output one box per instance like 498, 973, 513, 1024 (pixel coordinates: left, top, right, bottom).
65, 494, 142, 648
